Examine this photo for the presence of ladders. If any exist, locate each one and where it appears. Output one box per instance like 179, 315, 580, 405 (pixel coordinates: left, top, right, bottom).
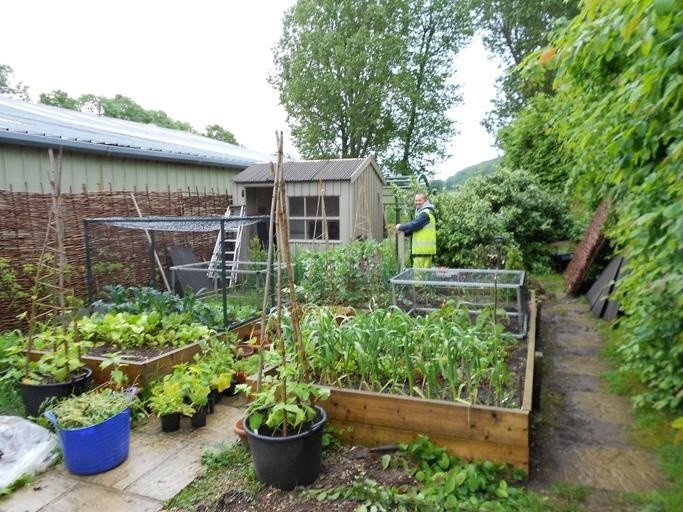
206, 205, 248, 287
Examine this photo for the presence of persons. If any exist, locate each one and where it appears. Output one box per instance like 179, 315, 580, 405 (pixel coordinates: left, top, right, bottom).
393, 193, 436, 288
